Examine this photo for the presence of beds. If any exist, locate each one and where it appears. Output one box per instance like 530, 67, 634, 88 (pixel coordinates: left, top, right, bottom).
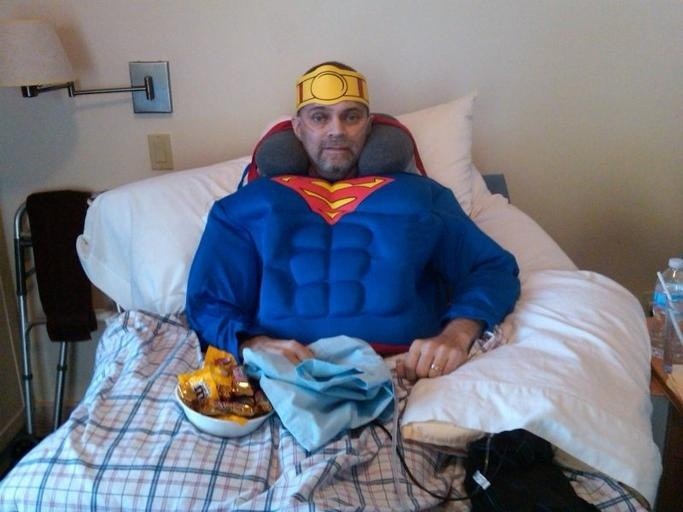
0, 176, 659, 511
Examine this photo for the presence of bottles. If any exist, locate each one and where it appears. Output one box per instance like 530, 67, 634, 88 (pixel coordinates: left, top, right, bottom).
650, 258, 683, 357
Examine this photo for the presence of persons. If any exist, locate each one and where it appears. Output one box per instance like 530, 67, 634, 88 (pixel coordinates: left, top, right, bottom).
186, 62, 522, 380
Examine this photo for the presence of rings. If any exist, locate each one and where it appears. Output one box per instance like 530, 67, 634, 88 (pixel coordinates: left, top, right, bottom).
430, 364, 441, 373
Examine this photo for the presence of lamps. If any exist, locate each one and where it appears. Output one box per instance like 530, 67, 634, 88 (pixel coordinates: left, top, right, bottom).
0, 22, 175, 116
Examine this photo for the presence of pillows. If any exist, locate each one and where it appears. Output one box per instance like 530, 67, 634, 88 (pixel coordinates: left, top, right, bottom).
77, 154, 254, 313
364, 92, 479, 212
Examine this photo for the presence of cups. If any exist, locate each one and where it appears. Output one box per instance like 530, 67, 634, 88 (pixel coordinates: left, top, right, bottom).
664, 291, 683, 364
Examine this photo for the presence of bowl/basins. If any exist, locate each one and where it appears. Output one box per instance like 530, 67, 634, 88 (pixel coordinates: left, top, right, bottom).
174, 380, 274, 438
